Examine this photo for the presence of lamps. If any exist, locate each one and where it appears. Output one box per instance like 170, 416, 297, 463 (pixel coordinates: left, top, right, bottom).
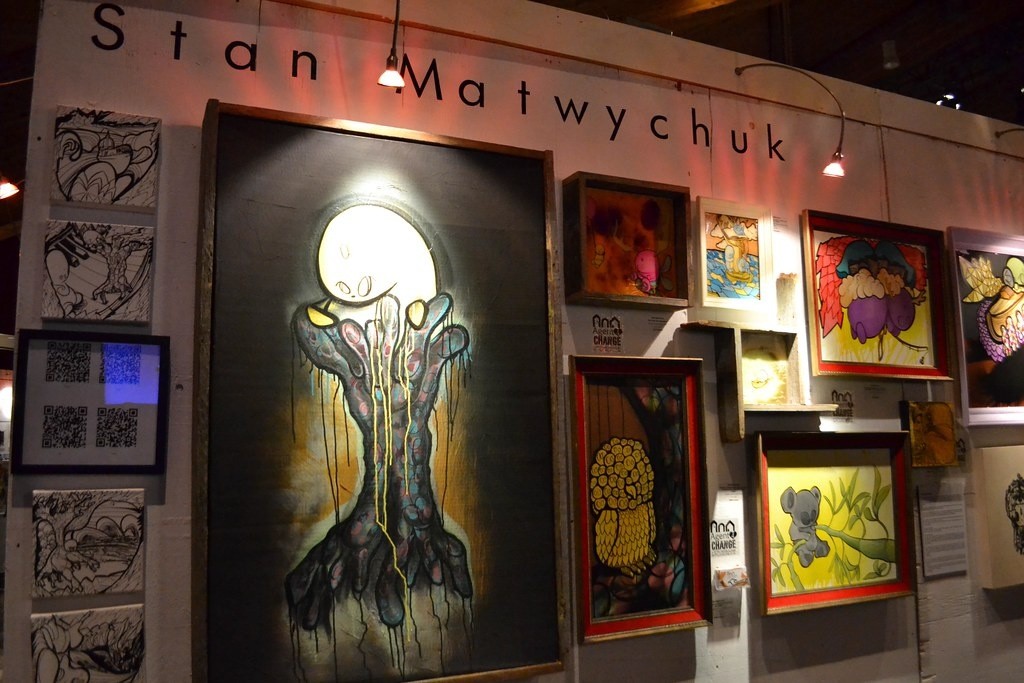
734, 62, 846, 178
378, 0, 405, 87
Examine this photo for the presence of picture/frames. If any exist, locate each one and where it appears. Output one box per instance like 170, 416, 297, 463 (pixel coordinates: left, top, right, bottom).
192, 98, 572, 683
744, 430, 920, 619
962, 425, 1023, 588
800, 208, 955, 383
567, 354, 715, 646
945, 223, 1024, 426
560, 170, 696, 312
687, 192, 778, 333
